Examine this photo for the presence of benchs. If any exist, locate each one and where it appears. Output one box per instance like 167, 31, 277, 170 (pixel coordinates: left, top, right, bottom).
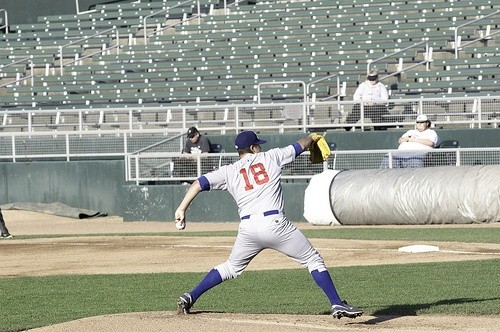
0, 0, 500, 131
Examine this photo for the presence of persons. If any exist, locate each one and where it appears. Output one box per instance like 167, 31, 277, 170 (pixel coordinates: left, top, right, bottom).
343, 70, 389, 131
380, 115, 439, 168
180, 126, 214, 186
0, 208, 15, 239
174, 131, 364, 319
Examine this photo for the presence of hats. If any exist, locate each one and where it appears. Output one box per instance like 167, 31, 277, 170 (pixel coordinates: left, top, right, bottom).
416, 115, 428, 122
235, 131, 267, 150
187, 127, 196, 137
367, 71, 378, 80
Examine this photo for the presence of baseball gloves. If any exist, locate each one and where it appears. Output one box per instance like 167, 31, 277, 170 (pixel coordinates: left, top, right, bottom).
309, 131, 331, 164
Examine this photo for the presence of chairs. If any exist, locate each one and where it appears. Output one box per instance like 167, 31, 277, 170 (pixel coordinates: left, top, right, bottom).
436, 140, 460, 148
210, 143, 223, 169
327, 142, 337, 170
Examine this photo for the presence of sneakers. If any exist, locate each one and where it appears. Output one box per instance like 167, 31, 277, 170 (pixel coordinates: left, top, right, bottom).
177, 293, 192, 314
331, 299, 364, 319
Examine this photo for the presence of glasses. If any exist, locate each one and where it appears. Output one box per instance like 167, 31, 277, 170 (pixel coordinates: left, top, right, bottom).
369, 79, 375, 81
416, 122, 424, 123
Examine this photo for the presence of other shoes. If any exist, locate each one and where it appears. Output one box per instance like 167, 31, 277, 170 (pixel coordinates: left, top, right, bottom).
0, 233, 13, 238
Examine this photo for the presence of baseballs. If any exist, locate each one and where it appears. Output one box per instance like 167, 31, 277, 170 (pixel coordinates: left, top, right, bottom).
176, 221, 184, 230
